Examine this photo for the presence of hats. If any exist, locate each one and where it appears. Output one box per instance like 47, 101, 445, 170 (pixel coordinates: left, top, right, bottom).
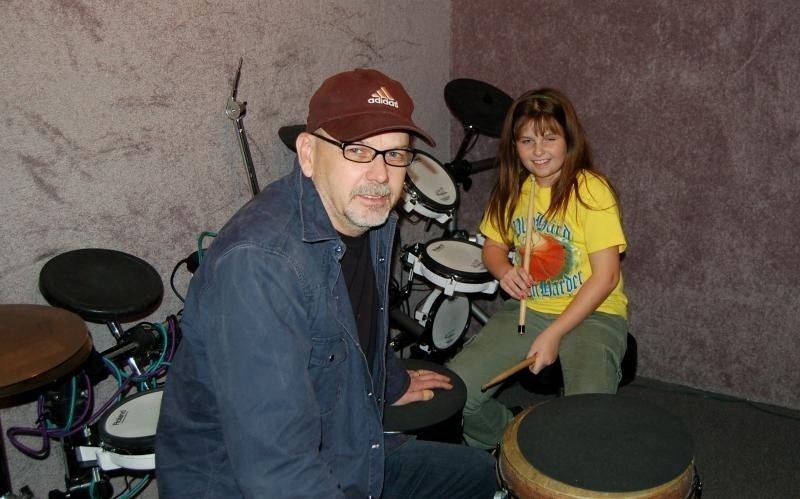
305, 68, 440, 148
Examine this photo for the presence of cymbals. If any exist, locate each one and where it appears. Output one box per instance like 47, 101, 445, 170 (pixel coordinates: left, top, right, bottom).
444, 79, 516, 138
0, 303, 94, 395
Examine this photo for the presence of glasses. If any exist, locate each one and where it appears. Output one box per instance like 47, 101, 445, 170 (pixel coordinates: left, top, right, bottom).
307, 132, 415, 168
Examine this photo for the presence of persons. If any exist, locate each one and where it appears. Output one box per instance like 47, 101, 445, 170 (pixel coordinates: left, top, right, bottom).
441, 87, 631, 448
154, 68, 501, 498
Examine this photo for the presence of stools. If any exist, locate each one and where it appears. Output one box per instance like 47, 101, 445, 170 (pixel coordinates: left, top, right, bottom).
514, 326, 641, 398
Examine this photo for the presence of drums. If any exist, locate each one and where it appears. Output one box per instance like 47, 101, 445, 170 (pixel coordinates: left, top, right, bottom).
500, 392, 700, 499
98, 384, 167, 471
416, 287, 470, 356
406, 238, 501, 297
401, 150, 460, 225
39, 248, 164, 323
